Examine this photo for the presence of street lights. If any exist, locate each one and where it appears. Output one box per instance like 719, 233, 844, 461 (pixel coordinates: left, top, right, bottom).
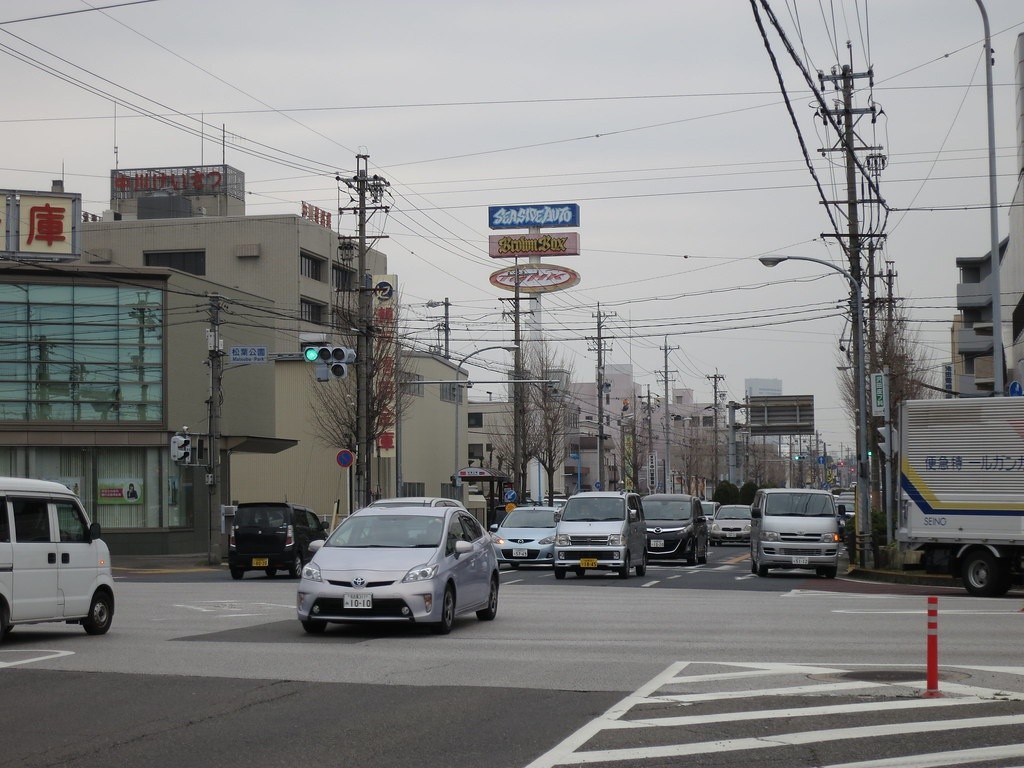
452, 345, 518, 492
598, 410, 635, 491
759, 252, 873, 574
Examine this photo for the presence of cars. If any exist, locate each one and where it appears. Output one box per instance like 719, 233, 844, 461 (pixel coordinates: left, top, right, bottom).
488, 506, 562, 569
294, 506, 499, 634
708, 505, 753, 546
329, 498, 470, 547
832, 491, 859, 527
543, 499, 568, 510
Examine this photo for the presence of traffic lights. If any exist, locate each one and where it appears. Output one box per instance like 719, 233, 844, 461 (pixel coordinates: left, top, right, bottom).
170, 435, 191, 461
794, 454, 806, 460
330, 363, 347, 379
869, 415, 886, 453
305, 346, 350, 363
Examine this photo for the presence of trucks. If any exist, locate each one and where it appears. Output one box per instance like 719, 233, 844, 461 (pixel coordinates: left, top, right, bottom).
893, 395, 1024, 597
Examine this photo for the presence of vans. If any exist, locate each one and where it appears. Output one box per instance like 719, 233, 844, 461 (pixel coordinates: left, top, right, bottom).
553, 491, 649, 579
640, 493, 708, 566
699, 502, 720, 544
228, 503, 332, 580
0, 475, 115, 647
750, 487, 846, 578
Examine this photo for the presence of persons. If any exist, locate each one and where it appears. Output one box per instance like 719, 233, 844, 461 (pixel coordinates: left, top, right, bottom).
426, 522, 450, 552
127, 483, 138, 500
71, 483, 79, 495
